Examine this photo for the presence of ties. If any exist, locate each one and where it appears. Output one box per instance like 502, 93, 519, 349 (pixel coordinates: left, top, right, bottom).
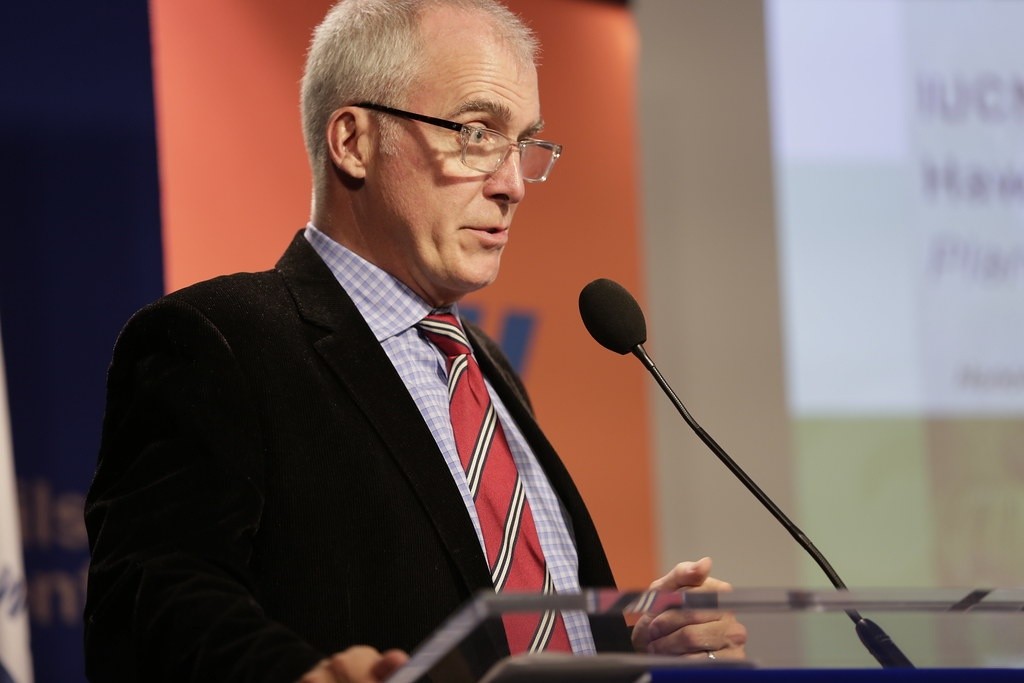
416, 311, 574, 657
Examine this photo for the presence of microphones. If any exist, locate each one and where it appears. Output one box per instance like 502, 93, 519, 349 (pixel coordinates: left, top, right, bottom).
577, 276, 920, 671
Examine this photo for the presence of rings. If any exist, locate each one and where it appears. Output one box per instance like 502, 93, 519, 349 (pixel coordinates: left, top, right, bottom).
706, 650, 717, 665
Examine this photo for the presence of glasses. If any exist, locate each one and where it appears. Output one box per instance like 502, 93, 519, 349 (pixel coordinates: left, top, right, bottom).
348, 102, 563, 184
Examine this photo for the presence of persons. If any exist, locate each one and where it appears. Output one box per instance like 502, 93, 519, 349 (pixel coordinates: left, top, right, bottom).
82, 0, 752, 683
